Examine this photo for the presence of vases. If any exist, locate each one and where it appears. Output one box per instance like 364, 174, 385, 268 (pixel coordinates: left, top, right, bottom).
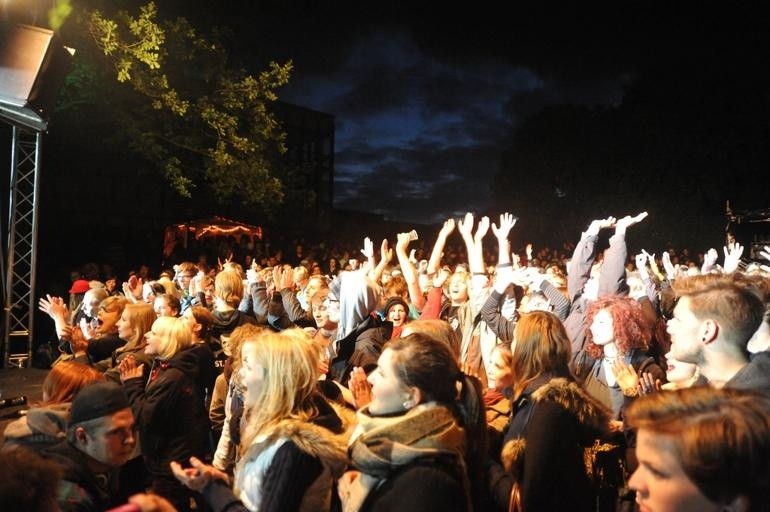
0, 21, 54, 108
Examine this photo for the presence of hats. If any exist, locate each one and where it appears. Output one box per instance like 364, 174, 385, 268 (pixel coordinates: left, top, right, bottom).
68, 280, 90, 294
71, 383, 128, 422
384, 297, 409, 320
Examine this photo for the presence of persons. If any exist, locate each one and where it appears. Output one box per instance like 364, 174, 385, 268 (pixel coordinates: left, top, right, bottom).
1, 212, 770, 511
